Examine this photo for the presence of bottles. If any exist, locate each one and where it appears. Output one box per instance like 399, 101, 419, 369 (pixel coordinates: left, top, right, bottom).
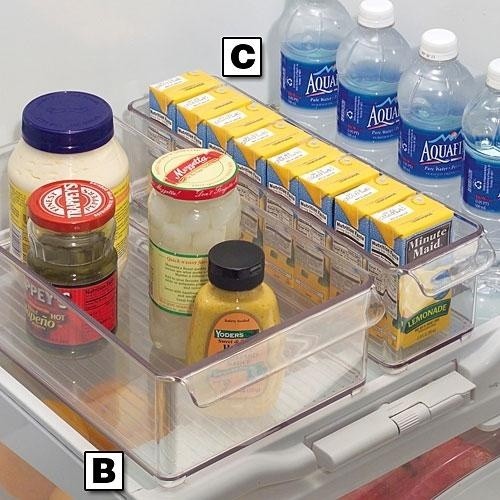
184, 239, 285, 418
147, 148, 242, 358
398, 30, 472, 206
337, 0, 409, 174
279, 1, 348, 141
462, 59, 500, 297
26, 180, 119, 358
8, 90, 128, 288
